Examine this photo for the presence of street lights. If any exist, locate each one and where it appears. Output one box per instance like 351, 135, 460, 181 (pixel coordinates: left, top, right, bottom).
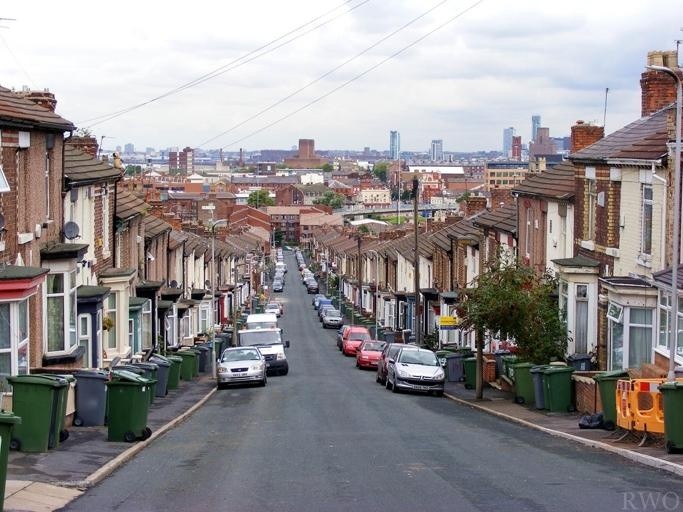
320, 253, 329, 295
210, 218, 229, 379
366, 248, 378, 339
644, 60, 683, 383
262, 240, 279, 289
250, 256, 257, 314
334, 256, 342, 316
232, 263, 248, 345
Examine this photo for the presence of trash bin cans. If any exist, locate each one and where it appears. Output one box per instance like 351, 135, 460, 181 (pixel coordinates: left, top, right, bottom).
72, 294, 265, 443
0, 408, 22, 512
657, 381, 683, 454
436, 343, 592, 413
592, 369, 630, 431
317, 277, 411, 344
5, 373, 75, 453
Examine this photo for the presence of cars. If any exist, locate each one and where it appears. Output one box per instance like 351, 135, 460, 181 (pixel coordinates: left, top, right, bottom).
335, 323, 448, 396
657, 330, 682, 356
294, 246, 319, 295
311, 293, 342, 328
283, 246, 292, 251
272, 247, 287, 292
613, 337, 623, 368
216, 299, 287, 390
18, 348, 25, 375
